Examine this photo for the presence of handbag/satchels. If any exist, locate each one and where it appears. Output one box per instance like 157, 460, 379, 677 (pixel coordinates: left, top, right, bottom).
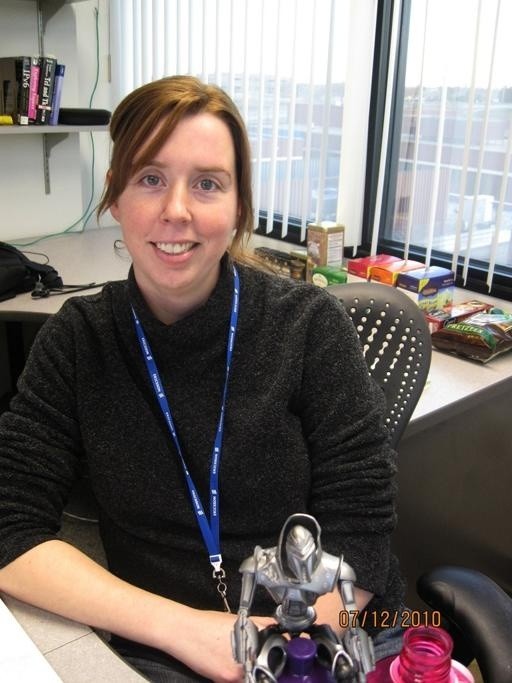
0, 240, 62, 302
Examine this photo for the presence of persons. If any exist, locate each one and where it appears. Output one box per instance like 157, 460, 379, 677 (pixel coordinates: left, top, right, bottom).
1, 76, 431, 683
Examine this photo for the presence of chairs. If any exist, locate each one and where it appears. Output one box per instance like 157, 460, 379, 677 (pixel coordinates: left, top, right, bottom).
322, 283, 512, 683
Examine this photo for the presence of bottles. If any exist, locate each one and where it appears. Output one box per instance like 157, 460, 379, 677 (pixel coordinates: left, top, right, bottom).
368, 621, 476, 682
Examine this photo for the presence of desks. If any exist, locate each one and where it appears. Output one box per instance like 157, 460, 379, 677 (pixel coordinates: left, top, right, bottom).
1, 224, 512, 621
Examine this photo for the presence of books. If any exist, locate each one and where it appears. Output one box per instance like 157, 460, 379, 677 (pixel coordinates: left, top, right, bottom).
0, 56, 66, 126
